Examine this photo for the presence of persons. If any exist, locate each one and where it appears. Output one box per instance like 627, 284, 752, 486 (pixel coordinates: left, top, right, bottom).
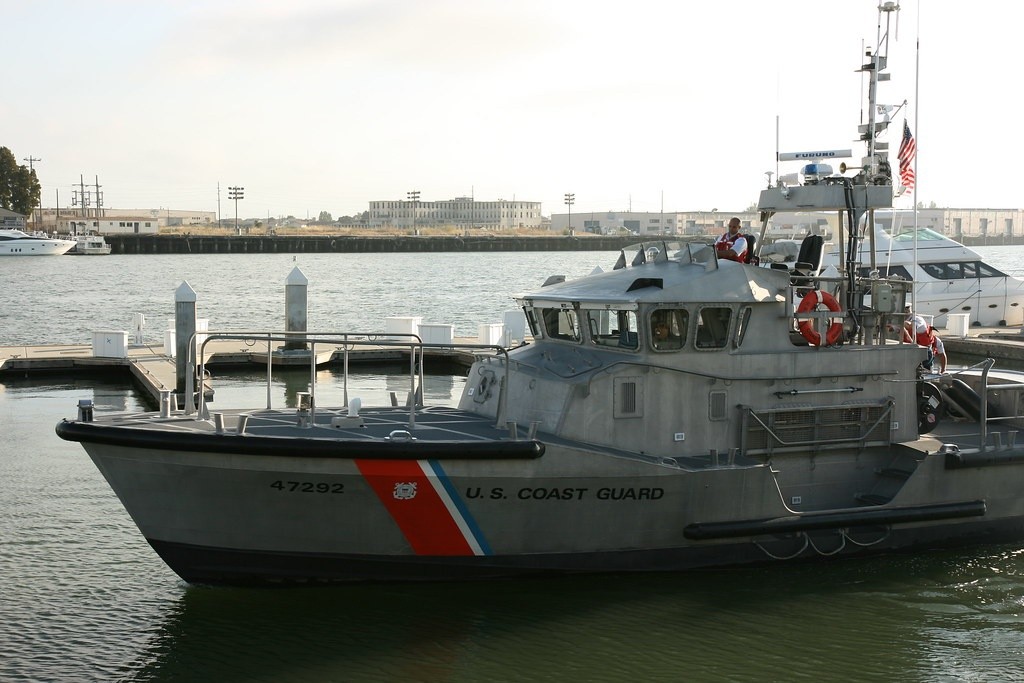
748, 255, 759, 264
888, 306, 933, 371
654, 323, 669, 337
713, 217, 748, 263
927, 335, 947, 372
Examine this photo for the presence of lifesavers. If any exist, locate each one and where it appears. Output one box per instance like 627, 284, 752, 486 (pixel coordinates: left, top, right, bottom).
796, 291, 845, 347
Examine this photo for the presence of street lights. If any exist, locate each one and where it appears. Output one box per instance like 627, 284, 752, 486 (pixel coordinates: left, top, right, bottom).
227, 185, 244, 234
407, 190, 422, 236
564, 193, 574, 235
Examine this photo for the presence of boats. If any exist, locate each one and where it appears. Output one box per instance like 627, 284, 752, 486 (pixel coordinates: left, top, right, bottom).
54, 0, 1023, 590
0, 227, 112, 256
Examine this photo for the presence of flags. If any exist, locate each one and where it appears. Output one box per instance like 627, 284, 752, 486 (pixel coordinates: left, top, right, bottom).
900, 165, 915, 195
897, 120, 916, 170
